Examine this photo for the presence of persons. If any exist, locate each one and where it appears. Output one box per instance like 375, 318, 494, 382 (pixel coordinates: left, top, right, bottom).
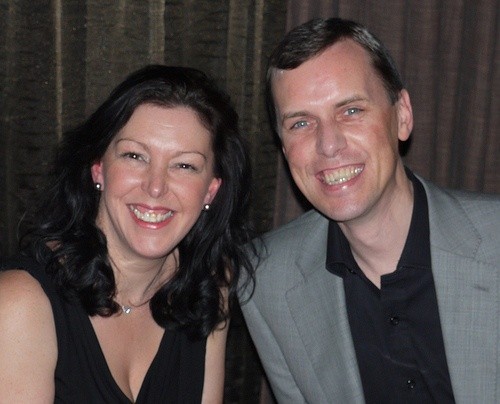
230, 15, 500, 404
2, 60, 271, 404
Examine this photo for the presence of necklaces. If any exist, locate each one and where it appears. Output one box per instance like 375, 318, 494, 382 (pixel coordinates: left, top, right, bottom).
111, 264, 154, 316
